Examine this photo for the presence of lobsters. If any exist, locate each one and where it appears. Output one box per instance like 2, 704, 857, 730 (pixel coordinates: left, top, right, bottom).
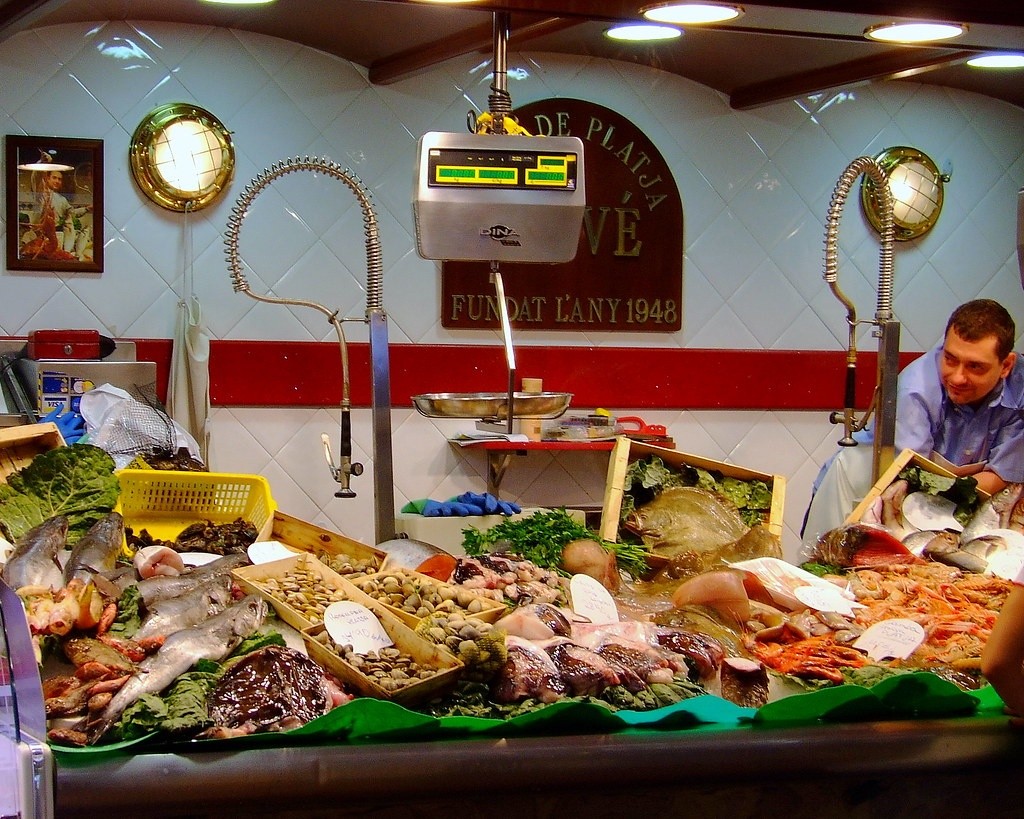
19, 177, 75, 260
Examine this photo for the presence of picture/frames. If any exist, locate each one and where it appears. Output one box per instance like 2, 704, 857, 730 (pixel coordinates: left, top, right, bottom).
6, 135, 104, 274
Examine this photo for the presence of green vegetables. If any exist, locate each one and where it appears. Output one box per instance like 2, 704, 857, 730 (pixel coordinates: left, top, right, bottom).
396, 663, 921, 720
0, 443, 286, 737
458, 502, 653, 581
897, 465, 979, 527
620, 452, 771, 542
797, 561, 845, 577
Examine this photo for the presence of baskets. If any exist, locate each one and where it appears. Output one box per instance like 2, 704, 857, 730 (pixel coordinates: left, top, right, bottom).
107, 467, 280, 570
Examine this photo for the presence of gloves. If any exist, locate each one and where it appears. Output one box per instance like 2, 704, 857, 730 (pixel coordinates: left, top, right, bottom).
399, 498, 483, 518
448, 491, 523, 516
38, 404, 85, 447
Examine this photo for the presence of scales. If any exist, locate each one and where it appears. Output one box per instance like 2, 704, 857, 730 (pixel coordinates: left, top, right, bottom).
410, 131, 587, 434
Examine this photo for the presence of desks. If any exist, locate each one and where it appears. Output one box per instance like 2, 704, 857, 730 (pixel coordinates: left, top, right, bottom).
447, 436, 676, 513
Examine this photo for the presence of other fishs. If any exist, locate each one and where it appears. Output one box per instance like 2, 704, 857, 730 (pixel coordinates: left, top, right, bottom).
2, 483, 1022, 747
63, 213, 89, 261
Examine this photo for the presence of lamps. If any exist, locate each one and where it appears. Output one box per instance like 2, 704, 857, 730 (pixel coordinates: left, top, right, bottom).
859, 146, 950, 242
129, 104, 236, 214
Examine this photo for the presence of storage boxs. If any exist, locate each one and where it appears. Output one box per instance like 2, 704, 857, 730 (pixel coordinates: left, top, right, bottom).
598, 437, 785, 574
112, 469, 509, 707
842, 448, 992, 527
0, 422, 67, 484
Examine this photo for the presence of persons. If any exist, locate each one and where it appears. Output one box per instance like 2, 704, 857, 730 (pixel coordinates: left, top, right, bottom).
800, 299, 1024, 541
30, 151, 93, 232
